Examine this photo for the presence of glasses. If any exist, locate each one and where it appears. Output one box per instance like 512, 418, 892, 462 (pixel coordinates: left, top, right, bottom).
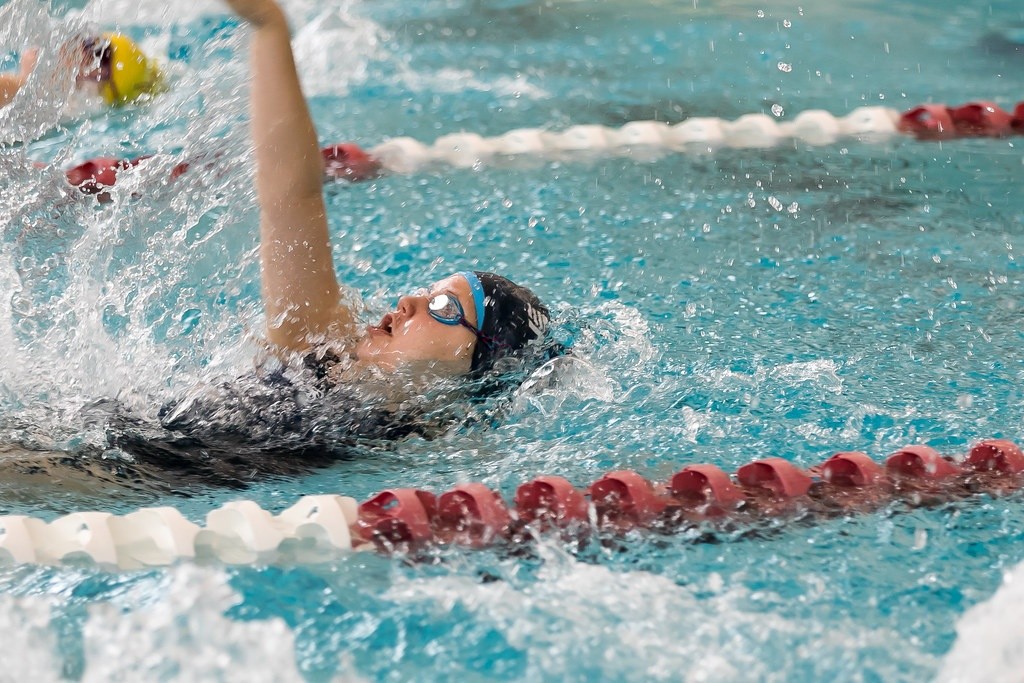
407, 285, 498, 351
81, 36, 120, 99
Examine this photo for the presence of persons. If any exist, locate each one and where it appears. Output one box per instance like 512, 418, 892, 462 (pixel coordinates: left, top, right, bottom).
228, 0, 548, 372
0, 31, 155, 110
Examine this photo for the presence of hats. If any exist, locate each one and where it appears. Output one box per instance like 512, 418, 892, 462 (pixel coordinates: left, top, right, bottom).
458, 268, 552, 379
506, 373, 506, 380
97, 32, 171, 107
